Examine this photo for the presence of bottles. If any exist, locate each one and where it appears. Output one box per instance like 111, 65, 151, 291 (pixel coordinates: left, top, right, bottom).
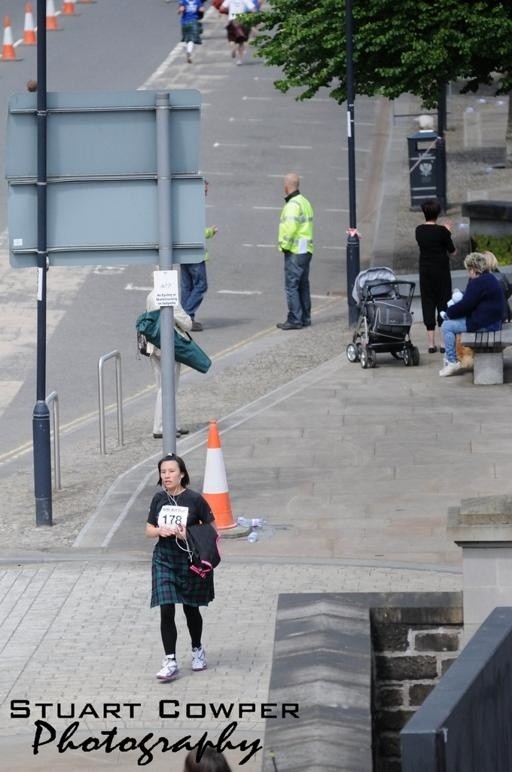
248, 530, 259, 544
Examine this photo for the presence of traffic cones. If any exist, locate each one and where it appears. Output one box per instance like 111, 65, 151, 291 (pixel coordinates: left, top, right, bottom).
46, 1, 97, 32
21, 1, 37, 46
1, 13, 23, 62
201, 417, 251, 539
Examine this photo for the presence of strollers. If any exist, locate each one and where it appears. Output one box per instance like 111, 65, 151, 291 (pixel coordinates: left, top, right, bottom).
345, 267, 423, 371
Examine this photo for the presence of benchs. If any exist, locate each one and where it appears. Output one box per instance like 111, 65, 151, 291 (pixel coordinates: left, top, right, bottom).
460, 296, 512, 384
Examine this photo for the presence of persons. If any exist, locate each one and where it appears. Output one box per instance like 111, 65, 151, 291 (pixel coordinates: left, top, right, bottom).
276, 173, 315, 330
144, 289, 194, 438
144, 455, 218, 681
414, 198, 512, 377
177, 0, 265, 66
26, 80, 37, 92
183, 747, 231, 772
181, 181, 220, 331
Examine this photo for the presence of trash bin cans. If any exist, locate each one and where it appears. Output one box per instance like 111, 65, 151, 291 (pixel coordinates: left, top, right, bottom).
405, 130, 449, 218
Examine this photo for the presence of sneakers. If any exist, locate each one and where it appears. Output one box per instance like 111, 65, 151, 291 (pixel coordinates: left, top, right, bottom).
156, 654, 178, 681
153, 426, 188, 439
190, 646, 206, 671
428, 345, 446, 354
276, 319, 312, 330
189, 319, 202, 333
439, 358, 463, 377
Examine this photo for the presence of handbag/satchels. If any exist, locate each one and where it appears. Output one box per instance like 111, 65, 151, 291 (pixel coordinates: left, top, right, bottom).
136, 308, 212, 374
